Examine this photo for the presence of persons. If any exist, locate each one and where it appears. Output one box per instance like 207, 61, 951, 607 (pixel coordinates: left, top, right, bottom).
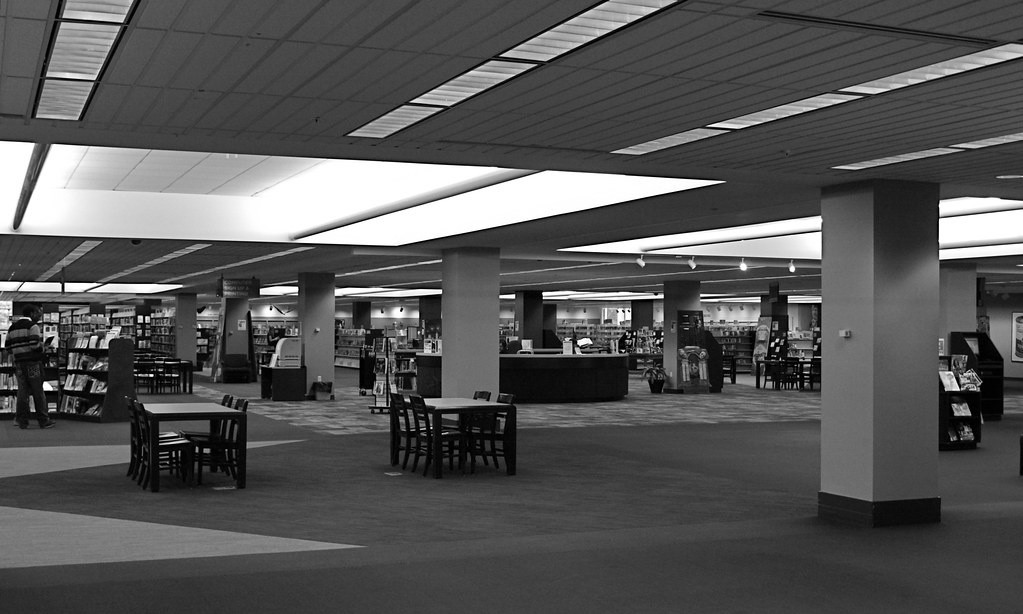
499, 341, 508, 354
267, 325, 279, 347
5, 304, 57, 429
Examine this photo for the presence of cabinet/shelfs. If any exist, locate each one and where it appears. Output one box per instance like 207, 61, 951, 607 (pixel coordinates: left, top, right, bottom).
334, 327, 377, 397
556, 320, 813, 374
0, 307, 299, 423
939, 356, 982, 451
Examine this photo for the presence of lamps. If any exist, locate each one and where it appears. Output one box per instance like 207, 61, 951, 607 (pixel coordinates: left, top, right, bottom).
687, 256, 696, 269
788, 260, 795, 273
739, 258, 748, 271
636, 255, 646, 268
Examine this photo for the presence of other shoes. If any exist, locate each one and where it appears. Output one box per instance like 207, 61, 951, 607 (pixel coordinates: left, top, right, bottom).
19, 424, 27, 429
40, 424, 56, 429
12, 417, 19, 425
50, 420, 57, 424
710, 386, 722, 393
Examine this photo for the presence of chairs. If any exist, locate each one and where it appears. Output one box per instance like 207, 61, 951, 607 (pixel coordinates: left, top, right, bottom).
762, 356, 821, 391
124, 394, 248, 491
390, 390, 513, 477
134, 353, 182, 396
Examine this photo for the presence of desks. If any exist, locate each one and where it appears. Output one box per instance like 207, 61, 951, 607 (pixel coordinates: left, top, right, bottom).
142, 402, 247, 493
260, 365, 306, 402
134, 360, 193, 395
390, 398, 517, 478
755, 359, 813, 392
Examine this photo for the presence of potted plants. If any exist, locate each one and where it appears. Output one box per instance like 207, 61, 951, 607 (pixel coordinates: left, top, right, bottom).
640, 362, 669, 394
